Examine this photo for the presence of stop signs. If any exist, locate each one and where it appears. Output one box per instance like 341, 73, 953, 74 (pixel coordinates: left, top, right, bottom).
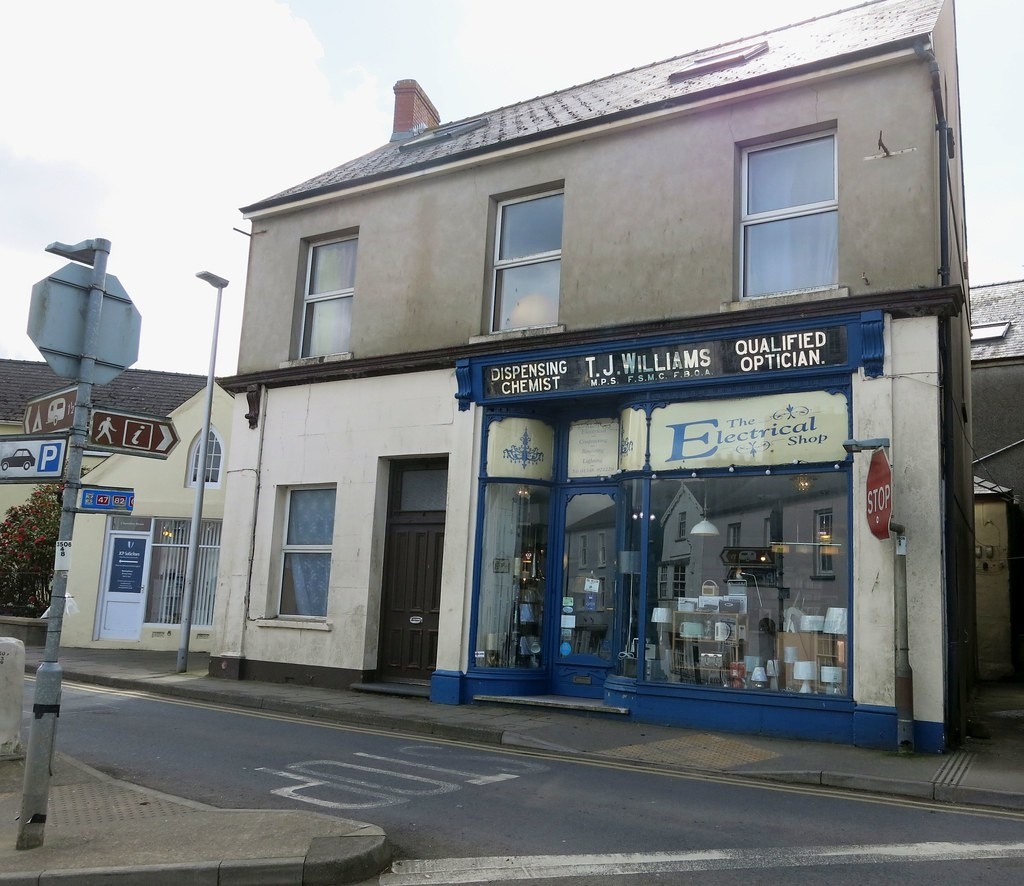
865, 444, 893, 543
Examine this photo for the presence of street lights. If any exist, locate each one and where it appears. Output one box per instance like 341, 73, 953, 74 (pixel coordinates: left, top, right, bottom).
174, 270, 232, 676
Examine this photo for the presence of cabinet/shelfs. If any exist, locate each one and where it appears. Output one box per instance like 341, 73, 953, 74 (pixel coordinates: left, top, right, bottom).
671, 610, 738, 673
774, 631, 848, 694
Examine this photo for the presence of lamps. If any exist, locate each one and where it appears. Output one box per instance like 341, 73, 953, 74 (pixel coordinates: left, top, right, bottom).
486, 474, 848, 695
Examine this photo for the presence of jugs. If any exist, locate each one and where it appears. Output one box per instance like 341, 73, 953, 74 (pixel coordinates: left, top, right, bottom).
714, 621, 731, 640
679, 622, 703, 637
